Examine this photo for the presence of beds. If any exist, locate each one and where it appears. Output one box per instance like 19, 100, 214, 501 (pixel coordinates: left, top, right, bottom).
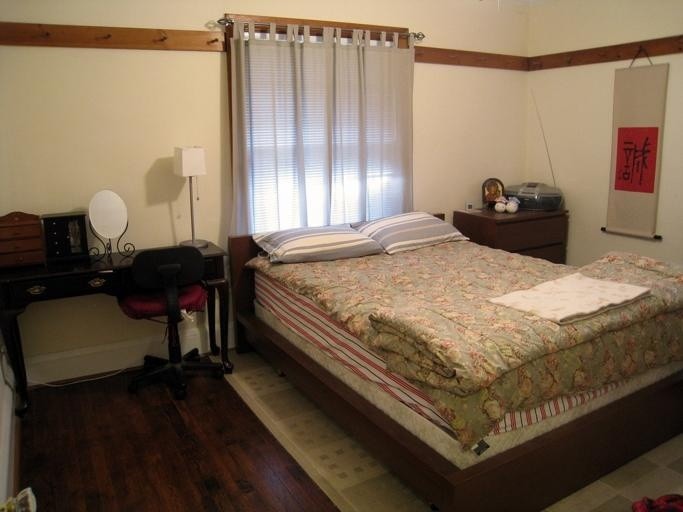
228, 210, 683, 512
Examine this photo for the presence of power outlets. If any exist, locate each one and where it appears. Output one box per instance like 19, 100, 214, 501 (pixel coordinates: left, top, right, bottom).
465, 203, 473, 210
3, 365, 16, 390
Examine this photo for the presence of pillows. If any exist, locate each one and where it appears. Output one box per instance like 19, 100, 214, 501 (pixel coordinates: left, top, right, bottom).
350, 211, 470, 254
252, 224, 387, 263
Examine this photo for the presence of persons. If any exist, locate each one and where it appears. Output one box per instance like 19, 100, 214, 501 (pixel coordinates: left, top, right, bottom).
485, 182, 500, 201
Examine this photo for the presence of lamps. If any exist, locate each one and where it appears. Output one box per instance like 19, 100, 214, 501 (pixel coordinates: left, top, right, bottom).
174, 146, 210, 249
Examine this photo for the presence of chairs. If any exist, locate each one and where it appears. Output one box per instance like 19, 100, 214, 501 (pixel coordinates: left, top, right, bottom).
116, 246, 226, 399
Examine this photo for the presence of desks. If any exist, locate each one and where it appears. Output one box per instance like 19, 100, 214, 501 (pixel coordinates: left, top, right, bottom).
0, 241, 236, 416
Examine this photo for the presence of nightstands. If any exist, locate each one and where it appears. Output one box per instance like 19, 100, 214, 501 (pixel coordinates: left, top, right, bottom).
454, 206, 568, 264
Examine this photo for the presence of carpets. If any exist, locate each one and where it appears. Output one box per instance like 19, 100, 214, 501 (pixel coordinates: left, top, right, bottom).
220, 351, 682, 512
